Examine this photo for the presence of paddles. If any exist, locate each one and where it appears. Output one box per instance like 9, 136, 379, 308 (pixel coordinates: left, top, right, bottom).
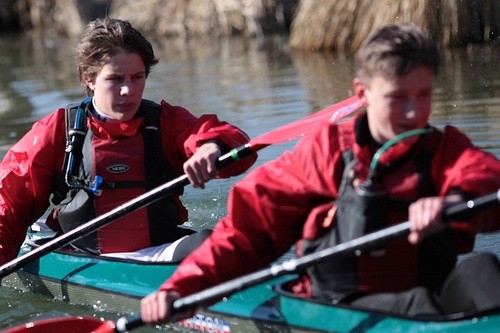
0, 93, 367, 281
0, 180, 500, 333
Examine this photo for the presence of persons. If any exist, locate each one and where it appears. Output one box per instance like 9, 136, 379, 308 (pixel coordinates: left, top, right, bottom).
0, 17, 258, 270
139, 22, 500, 327
